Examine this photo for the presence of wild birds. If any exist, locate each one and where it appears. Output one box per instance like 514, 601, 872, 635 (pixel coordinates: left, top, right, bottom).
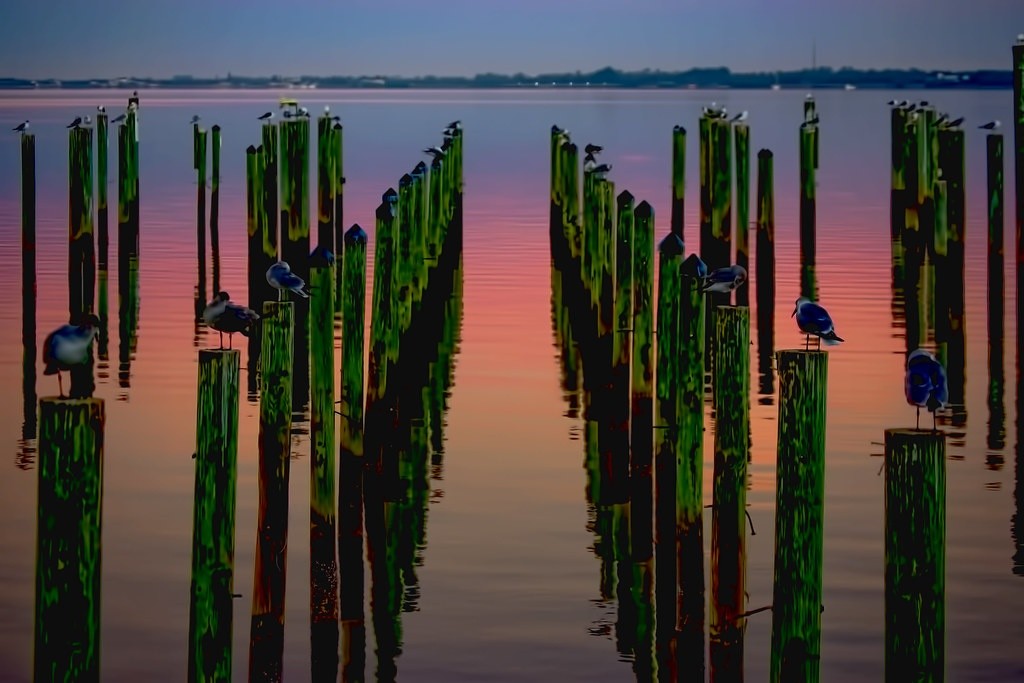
548, 123, 611, 175
97, 106, 106, 114
43, 313, 101, 400
203, 290, 271, 351
266, 260, 316, 302
127, 91, 140, 111
400, 120, 462, 185
889, 99, 965, 129
978, 119, 1001, 134
12, 119, 30, 134
111, 113, 128, 125
694, 261, 747, 307
258, 105, 332, 122
84, 115, 92, 127
791, 296, 845, 351
699, 105, 750, 125
67, 117, 81, 128
805, 114, 822, 128
189, 114, 201, 124
888, 347, 949, 432
